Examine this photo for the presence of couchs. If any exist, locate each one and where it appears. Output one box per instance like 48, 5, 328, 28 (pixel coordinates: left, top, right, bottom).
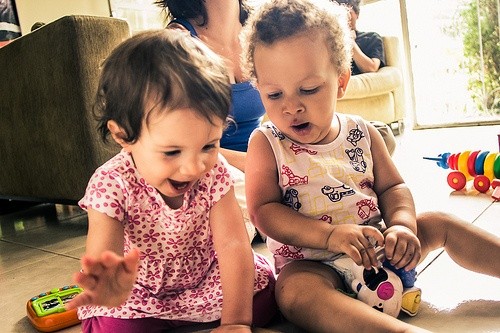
0, 15, 132, 210
322, 37, 405, 137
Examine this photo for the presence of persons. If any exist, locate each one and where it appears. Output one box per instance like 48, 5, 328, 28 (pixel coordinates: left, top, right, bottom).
152, 0, 266, 243
329, 1, 387, 78
240, 1, 500, 333
63, 24, 276, 333
